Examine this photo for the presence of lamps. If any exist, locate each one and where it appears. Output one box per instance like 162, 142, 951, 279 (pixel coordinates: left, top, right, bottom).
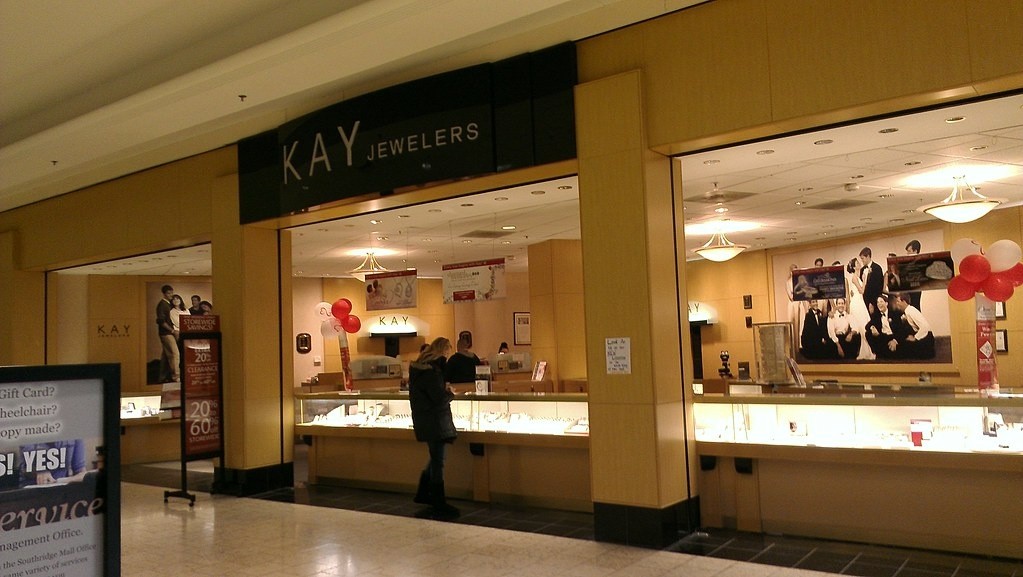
344, 253, 389, 282
695, 214, 748, 265
915, 174, 1007, 225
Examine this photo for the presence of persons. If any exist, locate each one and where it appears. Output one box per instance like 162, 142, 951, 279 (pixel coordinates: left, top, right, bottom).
446, 337, 482, 384
409, 337, 457, 505
156, 285, 191, 383
786, 258, 861, 360
499, 342, 509, 353
882, 240, 922, 318
865, 295, 936, 361
19, 438, 85, 486
367, 280, 383, 304
189, 295, 212, 316
420, 343, 430, 353
847, 247, 885, 359
63, 466, 104, 519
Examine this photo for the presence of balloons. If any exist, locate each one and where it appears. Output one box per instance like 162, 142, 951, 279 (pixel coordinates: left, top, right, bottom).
948, 238, 1023, 302
321, 318, 343, 337
341, 315, 361, 334
332, 299, 352, 319
315, 302, 334, 321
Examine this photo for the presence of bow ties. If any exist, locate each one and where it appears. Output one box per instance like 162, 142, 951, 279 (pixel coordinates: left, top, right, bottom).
881, 314, 885, 317
864, 266, 868, 269
839, 314, 844, 317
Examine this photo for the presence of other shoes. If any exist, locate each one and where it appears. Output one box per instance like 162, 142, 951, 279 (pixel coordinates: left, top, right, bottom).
430, 500, 460, 516
413, 495, 432, 504
170, 376, 181, 381
159, 377, 171, 383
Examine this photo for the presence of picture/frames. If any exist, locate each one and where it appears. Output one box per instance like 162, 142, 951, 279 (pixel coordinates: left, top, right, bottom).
766, 225, 962, 374
138, 274, 212, 391
742, 295, 753, 309
